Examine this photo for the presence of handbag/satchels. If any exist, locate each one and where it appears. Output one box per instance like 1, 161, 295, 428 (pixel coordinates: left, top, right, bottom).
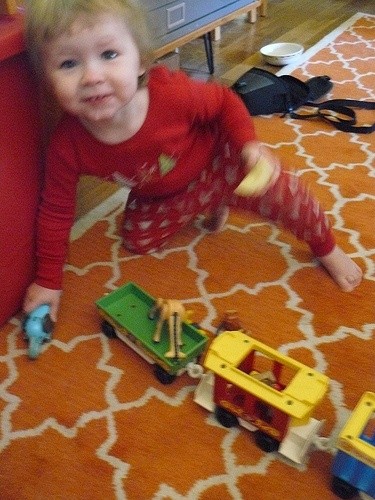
229, 67, 333, 116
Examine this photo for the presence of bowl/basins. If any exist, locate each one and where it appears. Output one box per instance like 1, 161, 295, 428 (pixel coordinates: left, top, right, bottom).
259, 43, 305, 65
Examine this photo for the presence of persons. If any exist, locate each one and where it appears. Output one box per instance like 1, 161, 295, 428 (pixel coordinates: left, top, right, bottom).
23, 0, 363, 323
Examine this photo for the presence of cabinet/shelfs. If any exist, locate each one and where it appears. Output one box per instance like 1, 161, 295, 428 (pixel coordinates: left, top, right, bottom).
140, 0, 262, 61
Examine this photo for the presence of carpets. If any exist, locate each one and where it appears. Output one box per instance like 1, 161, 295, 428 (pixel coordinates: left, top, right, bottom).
0, 12, 375, 500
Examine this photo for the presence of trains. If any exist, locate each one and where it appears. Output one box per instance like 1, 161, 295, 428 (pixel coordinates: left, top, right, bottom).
93, 281, 375, 500
